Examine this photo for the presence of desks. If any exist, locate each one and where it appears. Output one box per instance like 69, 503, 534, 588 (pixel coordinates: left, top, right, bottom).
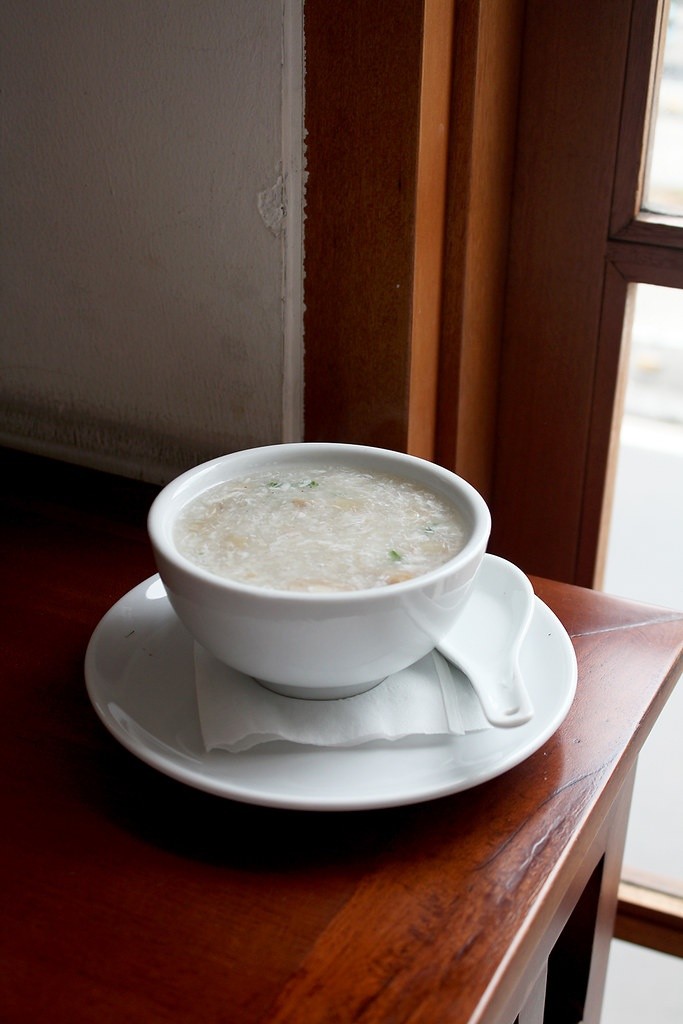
0, 450, 683, 1024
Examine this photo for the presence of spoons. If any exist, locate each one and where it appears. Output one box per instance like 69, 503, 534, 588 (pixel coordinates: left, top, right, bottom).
434, 552, 534, 728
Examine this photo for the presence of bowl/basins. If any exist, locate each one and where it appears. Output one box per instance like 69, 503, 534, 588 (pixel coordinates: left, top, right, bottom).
146, 442, 491, 701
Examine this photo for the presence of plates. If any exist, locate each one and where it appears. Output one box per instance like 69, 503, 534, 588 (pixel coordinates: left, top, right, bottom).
86, 573, 578, 811
